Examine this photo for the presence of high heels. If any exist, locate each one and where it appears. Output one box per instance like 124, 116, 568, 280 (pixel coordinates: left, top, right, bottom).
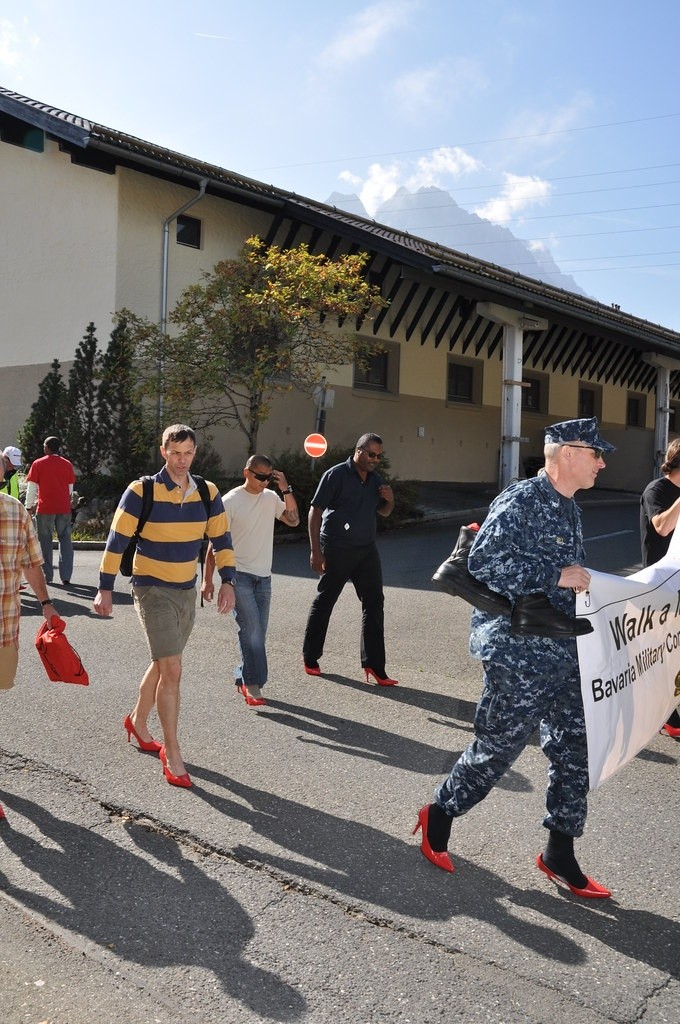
124, 713, 164, 751
365, 666, 398, 685
159, 746, 192, 787
412, 803, 455, 872
304, 658, 321, 675
537, 851, 612, 898
659, 723, 680, 738
238, 681, 266, 705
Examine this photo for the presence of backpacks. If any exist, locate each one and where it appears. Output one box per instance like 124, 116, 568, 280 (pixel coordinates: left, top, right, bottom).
119, 474, 209, 577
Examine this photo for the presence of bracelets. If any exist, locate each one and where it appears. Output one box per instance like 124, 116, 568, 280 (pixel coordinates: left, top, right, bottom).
40, 599, 52, 607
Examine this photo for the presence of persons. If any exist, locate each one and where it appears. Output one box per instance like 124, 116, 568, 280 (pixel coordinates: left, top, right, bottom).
94, 423, 236, 788
0, 446, 60, 821
24, 436, 76, 586
201, 453, 300, 705
303, 431, 398, 686
640, 437, 680, 739
411, 416, 616, 899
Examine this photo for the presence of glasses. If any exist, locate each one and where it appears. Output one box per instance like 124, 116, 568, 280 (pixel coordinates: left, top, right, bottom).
247, 469, 273, 482
359, 446, 383, 460
563, 444, 605, 459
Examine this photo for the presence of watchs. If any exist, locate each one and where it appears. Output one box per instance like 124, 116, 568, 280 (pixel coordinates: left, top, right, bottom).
280, 485, 293, 495
222, 578, 236, 586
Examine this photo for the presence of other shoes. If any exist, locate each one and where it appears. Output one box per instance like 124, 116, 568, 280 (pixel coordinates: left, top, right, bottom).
18, 584, 27, 589
62, 580, 71, 585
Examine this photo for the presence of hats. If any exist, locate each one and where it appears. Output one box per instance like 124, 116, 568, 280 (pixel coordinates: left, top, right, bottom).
4, 446, 22, 466
545, 416, 615, 453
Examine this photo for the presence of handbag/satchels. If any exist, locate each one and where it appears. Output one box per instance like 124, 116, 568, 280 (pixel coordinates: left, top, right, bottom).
35, 614, 90, 686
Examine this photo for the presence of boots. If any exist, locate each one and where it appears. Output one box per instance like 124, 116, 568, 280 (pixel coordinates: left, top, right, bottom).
430, 525, 512, 615
510, 591, 594, 636
